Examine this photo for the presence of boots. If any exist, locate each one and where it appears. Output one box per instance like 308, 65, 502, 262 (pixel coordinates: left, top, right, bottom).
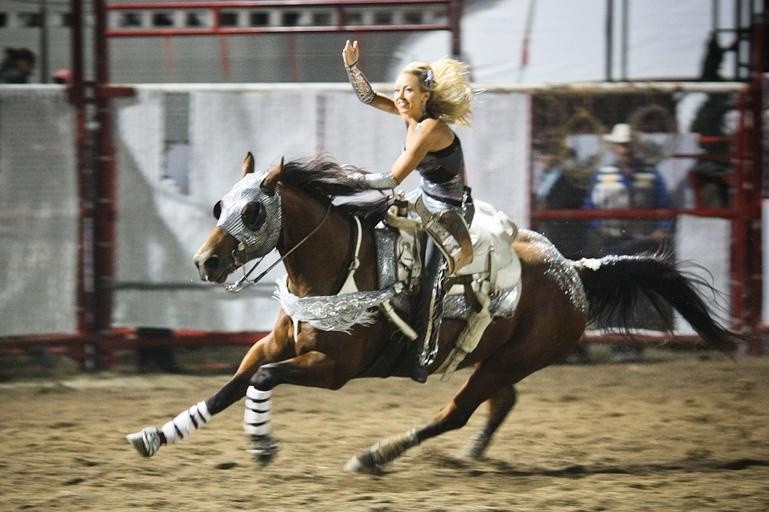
411, 365, 427, 383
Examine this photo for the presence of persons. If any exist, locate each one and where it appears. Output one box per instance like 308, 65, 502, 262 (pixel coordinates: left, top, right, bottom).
341, 38, 478, 384
534, 123, 677, 255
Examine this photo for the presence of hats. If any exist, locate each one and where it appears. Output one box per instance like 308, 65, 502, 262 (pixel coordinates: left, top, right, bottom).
602, 123, 636, 144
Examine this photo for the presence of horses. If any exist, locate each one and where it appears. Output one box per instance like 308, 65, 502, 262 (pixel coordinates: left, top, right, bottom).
125, 151, 749, 475
